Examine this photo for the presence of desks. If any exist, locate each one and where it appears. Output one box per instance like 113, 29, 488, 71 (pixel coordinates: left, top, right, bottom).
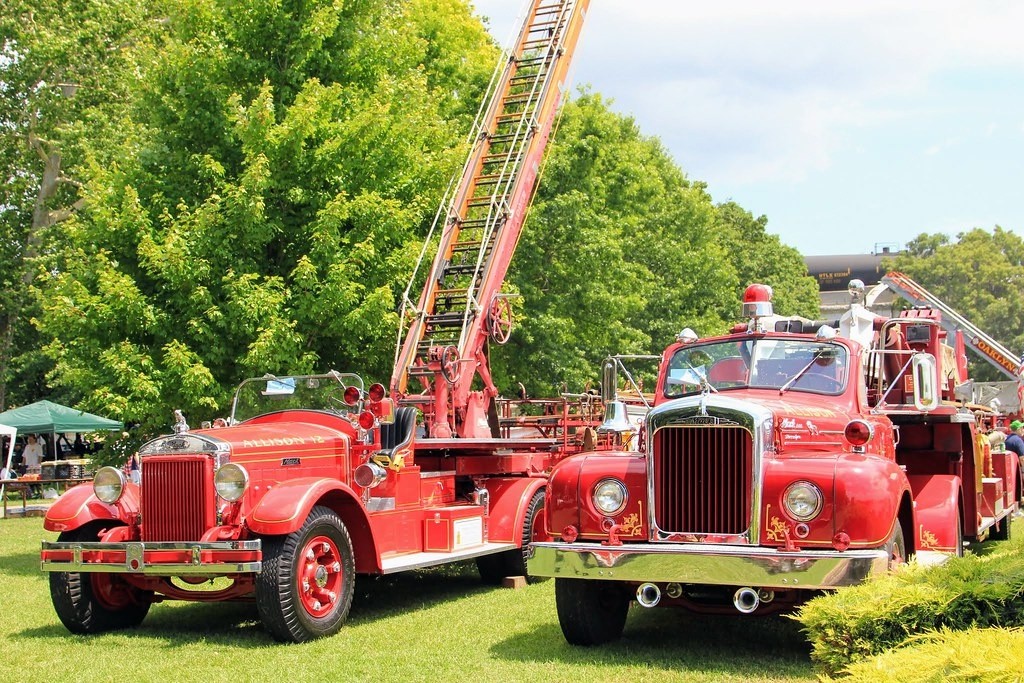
0, 477, 94, 519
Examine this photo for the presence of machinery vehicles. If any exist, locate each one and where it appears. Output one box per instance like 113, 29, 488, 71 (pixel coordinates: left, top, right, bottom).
39, 0, 653, 644
527, 272, 1024, 647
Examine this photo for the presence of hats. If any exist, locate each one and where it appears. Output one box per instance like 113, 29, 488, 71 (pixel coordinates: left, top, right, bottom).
1009, 420, 1024, 430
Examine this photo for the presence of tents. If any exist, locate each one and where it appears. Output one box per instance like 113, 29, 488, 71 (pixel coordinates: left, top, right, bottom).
0, 400, 124, 502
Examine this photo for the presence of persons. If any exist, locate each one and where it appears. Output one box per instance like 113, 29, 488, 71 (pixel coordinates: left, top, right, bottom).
121, 418, 230, 483
1, 434, 43, 498
1004, 420, 1024, 507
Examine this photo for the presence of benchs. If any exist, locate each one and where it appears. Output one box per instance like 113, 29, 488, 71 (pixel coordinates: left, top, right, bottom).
324, 406, 418, 462
709, 356, 839, 392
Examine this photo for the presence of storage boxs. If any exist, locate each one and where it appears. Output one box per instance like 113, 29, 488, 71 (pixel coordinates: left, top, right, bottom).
7, 505, 48, 518
56, 462, 84, 479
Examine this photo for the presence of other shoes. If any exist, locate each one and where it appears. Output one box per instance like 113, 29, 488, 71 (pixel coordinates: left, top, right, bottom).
1014, 510, 1024, 517
